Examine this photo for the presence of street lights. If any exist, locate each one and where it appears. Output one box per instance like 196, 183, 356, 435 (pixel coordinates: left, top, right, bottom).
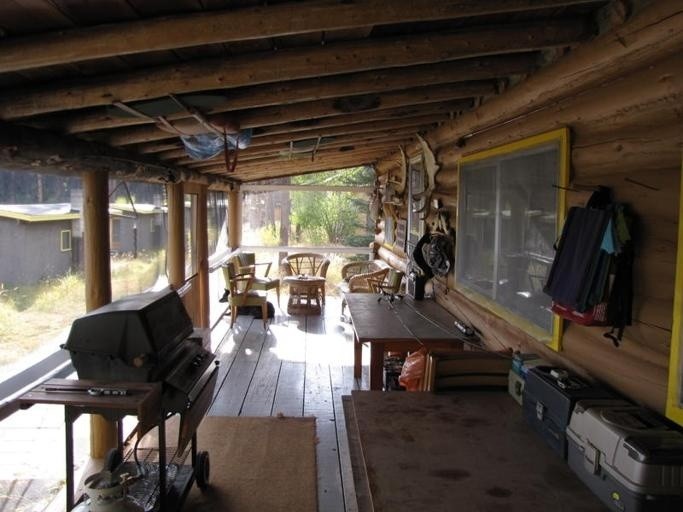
132, 221, 137, 258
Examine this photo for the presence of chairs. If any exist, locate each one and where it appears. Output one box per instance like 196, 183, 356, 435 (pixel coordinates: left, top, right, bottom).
341, 262, 404, 327
280, 252, 330, 307
421, 347, 516, 394
221, 259, 268, 331
231, 252, 280, 309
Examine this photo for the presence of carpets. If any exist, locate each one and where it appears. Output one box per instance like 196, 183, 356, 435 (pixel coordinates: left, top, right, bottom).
121, 410, 323, 510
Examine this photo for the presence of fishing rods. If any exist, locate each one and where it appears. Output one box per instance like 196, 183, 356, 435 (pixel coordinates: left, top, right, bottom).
378, 286, 515, 359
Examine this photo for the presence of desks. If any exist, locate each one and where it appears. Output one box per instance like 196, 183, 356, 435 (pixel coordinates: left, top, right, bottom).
282, 276, 326, 316
341, 292, 483, 392
339, 384, 614, 512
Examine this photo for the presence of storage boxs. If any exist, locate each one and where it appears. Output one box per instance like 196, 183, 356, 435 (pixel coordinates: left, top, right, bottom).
505, 357, 555, 403
562, 390, 682, 512
519, 363, 627, 460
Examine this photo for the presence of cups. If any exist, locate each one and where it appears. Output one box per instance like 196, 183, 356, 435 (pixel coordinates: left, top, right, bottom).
83, 471, 125, 512
